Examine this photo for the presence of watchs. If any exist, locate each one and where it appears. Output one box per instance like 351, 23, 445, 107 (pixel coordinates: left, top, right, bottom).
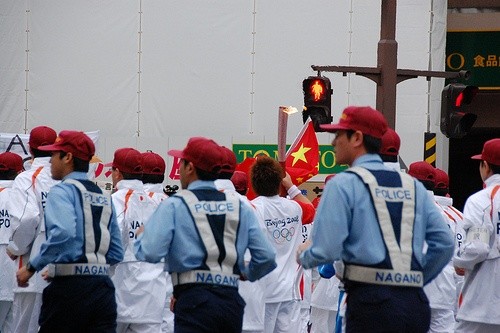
26, 262, 36, 273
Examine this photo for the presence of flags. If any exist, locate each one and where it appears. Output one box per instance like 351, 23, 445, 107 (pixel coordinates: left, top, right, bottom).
281, 116, 320, 197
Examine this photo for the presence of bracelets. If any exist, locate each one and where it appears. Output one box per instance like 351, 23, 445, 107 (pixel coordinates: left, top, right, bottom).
287, 185, 302, 199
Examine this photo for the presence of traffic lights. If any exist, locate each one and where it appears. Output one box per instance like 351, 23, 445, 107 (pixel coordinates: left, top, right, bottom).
301, 76, 334, 132
440, 82, 480, 140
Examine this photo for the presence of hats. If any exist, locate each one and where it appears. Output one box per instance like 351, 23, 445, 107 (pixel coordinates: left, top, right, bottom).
434, 168, 449, 189
29, 126, 56, 148
379, 127, 400, 155
231, 171, 248, 191
218, 146, 237, 173
0, 152, 23, 171
38, 130, 95, 161
111, 148, 144, 174
168, 137, 226, 174
471, 138, 500, 165
140, 152, 165, 174
408, 161, 438, 183
319, 106, 389, 139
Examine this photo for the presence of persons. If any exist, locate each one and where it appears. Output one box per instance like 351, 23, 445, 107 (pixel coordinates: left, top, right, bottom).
0, 106, 500, 333
